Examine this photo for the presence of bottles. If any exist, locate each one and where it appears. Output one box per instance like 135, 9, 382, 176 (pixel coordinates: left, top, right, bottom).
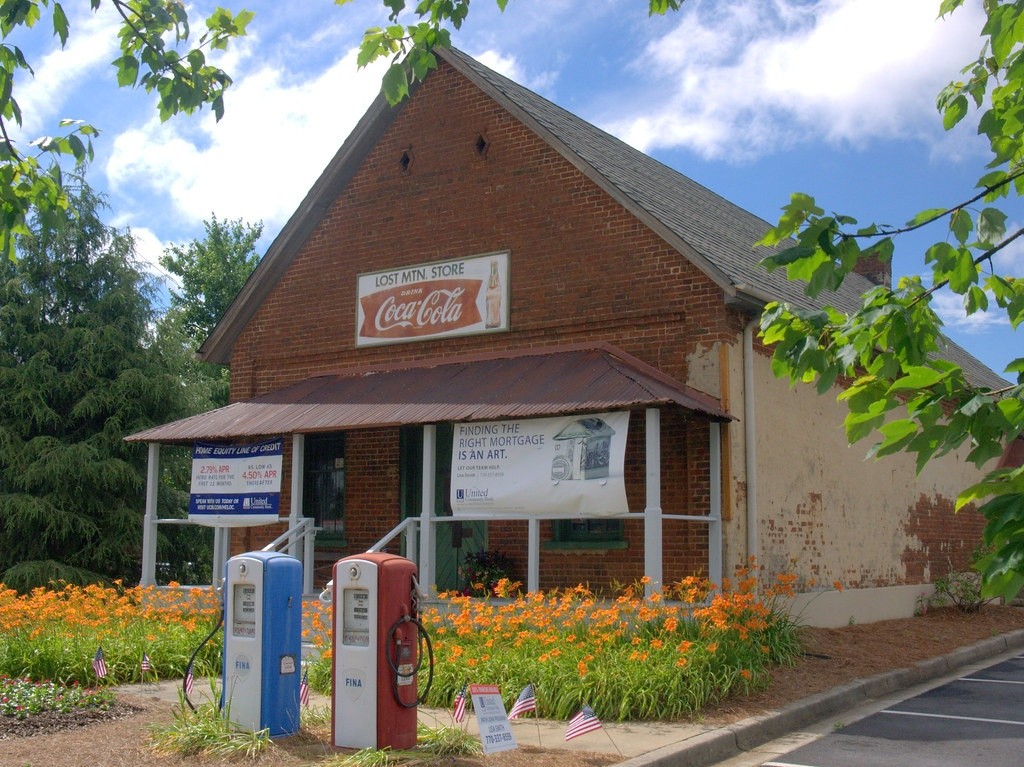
486, 260, 501, 327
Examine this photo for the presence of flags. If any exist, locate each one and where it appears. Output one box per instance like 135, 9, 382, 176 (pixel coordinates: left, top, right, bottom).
299, 669, 309, 706
565, 705, 602, 741
508, 684, 536, 721
92, 646, 108, 677
141, 651, 150, 671
454, 678, 469, 723
186, 663, 193, 693
